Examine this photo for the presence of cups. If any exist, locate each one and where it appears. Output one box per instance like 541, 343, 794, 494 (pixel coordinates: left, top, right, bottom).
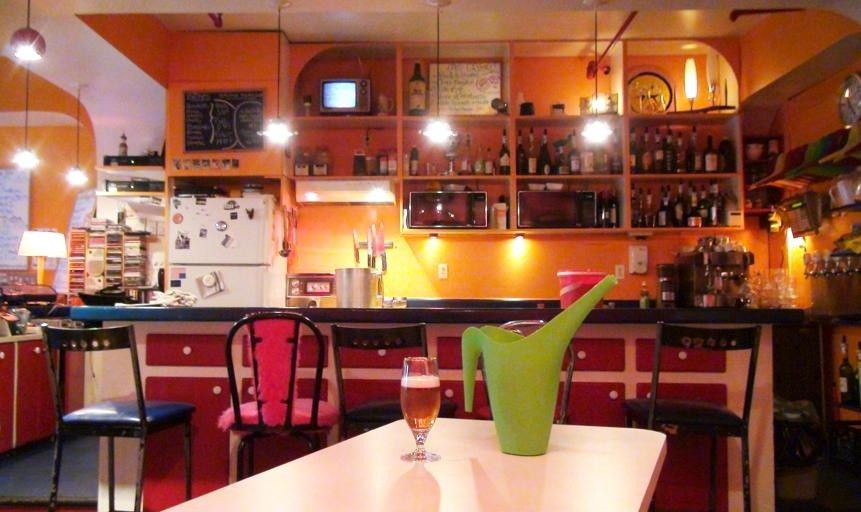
335, 267, 370, 309
458, 272, 619, 458
399, 356, 441, 461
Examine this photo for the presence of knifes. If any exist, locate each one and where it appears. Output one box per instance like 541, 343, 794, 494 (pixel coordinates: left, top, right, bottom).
353, 228, 359, 262
378, 222, 387, 270
367, 229, 371, 268
371, 224, 377, 268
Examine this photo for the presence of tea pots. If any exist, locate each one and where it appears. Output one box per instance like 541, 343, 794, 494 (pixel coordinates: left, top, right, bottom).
829, 179, 861, 206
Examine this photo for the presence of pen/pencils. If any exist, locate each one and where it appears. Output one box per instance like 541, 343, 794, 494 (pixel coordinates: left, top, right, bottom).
350, 222, 387, 272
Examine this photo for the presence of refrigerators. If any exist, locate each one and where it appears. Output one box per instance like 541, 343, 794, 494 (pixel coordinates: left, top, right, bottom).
167, 194, 291, 308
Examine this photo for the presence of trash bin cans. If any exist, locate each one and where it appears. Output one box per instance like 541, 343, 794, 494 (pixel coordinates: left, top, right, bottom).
773, 418, 821, 500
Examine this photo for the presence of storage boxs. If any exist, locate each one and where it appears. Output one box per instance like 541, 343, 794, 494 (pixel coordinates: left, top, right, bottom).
558, 272, 605, 308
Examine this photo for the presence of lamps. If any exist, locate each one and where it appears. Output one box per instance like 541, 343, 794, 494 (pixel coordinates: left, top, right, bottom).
18, 232, 67, 288
419, 2, 459, 146
14, 64, 43, 168
582, 5, 613, 147
10, 3, 47, 63
64, 88, 90, 188
259, 12, 299, 146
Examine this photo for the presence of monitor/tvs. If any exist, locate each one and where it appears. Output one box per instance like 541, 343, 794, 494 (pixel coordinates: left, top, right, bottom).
316, 78, 372, 112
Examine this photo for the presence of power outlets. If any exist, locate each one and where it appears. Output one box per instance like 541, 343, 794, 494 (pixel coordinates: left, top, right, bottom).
616, 265, 625, 279
438, 264, 447, 278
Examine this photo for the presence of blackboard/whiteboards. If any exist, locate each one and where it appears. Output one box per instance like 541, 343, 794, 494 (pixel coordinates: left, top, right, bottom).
183, 87, 266, 152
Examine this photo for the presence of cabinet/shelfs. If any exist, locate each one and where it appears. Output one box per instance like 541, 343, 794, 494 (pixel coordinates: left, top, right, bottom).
69, 301, 803, 512
1, 332, 84, 454
165, 30, 786, 301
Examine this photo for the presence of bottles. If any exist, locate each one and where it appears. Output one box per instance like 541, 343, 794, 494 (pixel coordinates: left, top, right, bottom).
424, 160, 438, 176
719, 136, 734, 173
557, 145, 568, 174
687, 194, 702, 227
640, 282, 648, 308
403, 153, 408, 176
660, 276, 675, 309
804, 256, 860, 317
527, 129, 538, 175
629, 127, 638, 172
376, 148, 388, 176
658, 199, 672, 226
675, 180, 686, 226
582, 196, 594, 226
602, 148, 612, 174
474, 146, 485, 176
597, 193, 605, 227
569, 135, 579, 174
662, 186, 672, 208
652, 129, 663, 173
608, 189, 617, 226
500, 129, 511, 174
303, 95, 312, 116
312, 149, 329, 175
353, 154, 366, 176
484, 148, 493, 175
388, 148, 398, 176
630, 184, 638, 226
515, 129, 525, 175
461, 134, 474, 174
663, 329, 738, 348
294, 150, 308, 175
704, 133, 718, 173
722, 189, 737, 225
704, 267, 715, 308
119, 133, 128, 157
242, 184, 264, 198
516, 91, 525, 115
664, 130, 674, 173
853, 344, 861, 406
644, 189, 656, 228
710, 179, 721, 226
639, 128, 651, 172
639, 189, 643, 227
408, 62, 425, 116
675, 131, 687, 172
699, 186, 709, 225
365, 157, 375, 175
714, 270, 795, 308
581, 149, 593, 174
410, 145, 419, 176
688, 126, 702, 172
840, 336, 854, 407
520, 102, 534, 116
552, 104, 564, 114
540, 129, 551, 175
613, 154, 620, 173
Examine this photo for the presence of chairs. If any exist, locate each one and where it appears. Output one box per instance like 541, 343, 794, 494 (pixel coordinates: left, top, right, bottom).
41, 320, 193, 511
623, 320, 764, 511
485, 320, 575, 425
327, 325, 458, 433
220, 314, 339, 480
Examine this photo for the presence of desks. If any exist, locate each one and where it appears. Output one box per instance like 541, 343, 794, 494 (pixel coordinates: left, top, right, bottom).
158, 417, 666, 511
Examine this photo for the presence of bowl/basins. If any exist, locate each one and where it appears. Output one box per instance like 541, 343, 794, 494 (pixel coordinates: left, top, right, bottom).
546, 182, 563, 190
443, 184, 466, 191
528, 184, 545, 191
